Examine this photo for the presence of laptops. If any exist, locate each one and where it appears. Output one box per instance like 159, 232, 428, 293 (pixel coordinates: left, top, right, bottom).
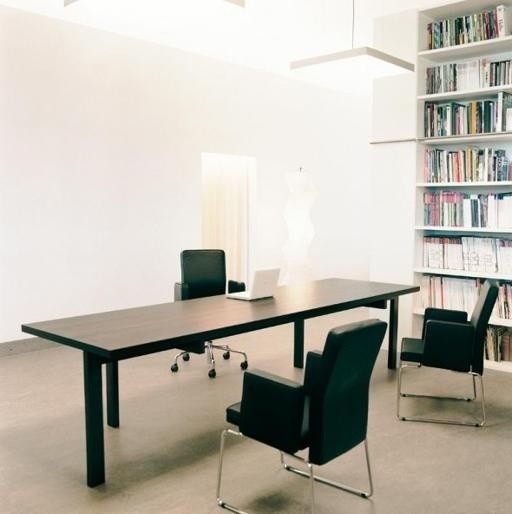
226, 268, 281, 300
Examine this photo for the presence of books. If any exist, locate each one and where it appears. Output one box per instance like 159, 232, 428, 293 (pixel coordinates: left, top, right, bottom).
420, 3, 511, 363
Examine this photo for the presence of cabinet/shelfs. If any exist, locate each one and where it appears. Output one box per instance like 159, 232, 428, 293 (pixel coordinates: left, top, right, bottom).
412, 0, 512, 400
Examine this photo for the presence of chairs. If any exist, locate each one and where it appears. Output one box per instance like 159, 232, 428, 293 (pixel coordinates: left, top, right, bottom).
399, 279, 499, 426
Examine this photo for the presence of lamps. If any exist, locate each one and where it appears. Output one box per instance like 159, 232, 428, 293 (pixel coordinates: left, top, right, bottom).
287, 0, 416, 85
